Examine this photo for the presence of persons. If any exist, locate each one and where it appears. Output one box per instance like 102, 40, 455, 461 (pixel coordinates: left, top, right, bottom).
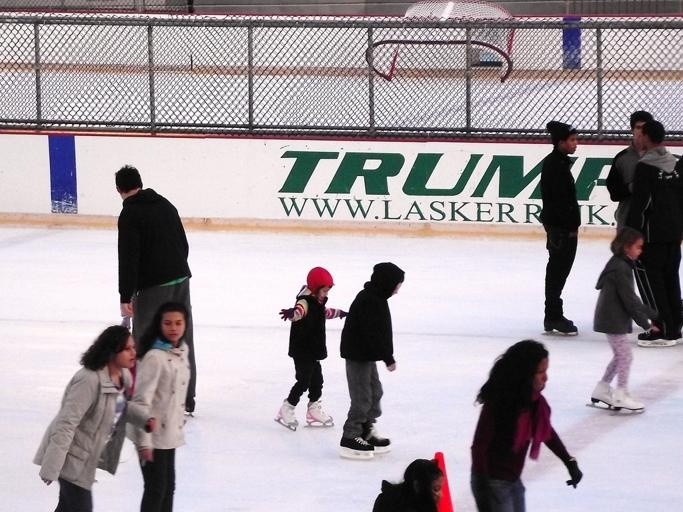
279, 267, 349, 425
115, 165, 195, 413
372, 459, 444, 512
590, 227, 660, 410
33, 326, 157, 512
340, 261, 405, 453
540, 121, 581, 332
470, 340, 583, 511
123, 301, 191, 511
606, 111, 655, 232
626, 120, 682, 342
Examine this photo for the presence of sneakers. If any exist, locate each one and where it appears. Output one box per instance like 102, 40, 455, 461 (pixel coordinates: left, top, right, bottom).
613, 389, 645, 410
305, 402, 333, 423
279, 399, 298, 425
638, 330, 682, 345
544, 316, 577, 332
362, 432, 390, 446
185, 395, 195, 412
340, 436, 374, 452
592, 383, 612, 404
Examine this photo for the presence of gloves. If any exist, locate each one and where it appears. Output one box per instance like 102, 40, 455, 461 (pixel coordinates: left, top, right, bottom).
339, 309, 348, 318
566, 461, 583, 488
279, 307, 298, 321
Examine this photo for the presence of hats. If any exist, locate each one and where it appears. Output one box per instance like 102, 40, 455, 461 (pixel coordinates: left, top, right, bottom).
547, 121, 578, 144
307, 267, 333, 290
